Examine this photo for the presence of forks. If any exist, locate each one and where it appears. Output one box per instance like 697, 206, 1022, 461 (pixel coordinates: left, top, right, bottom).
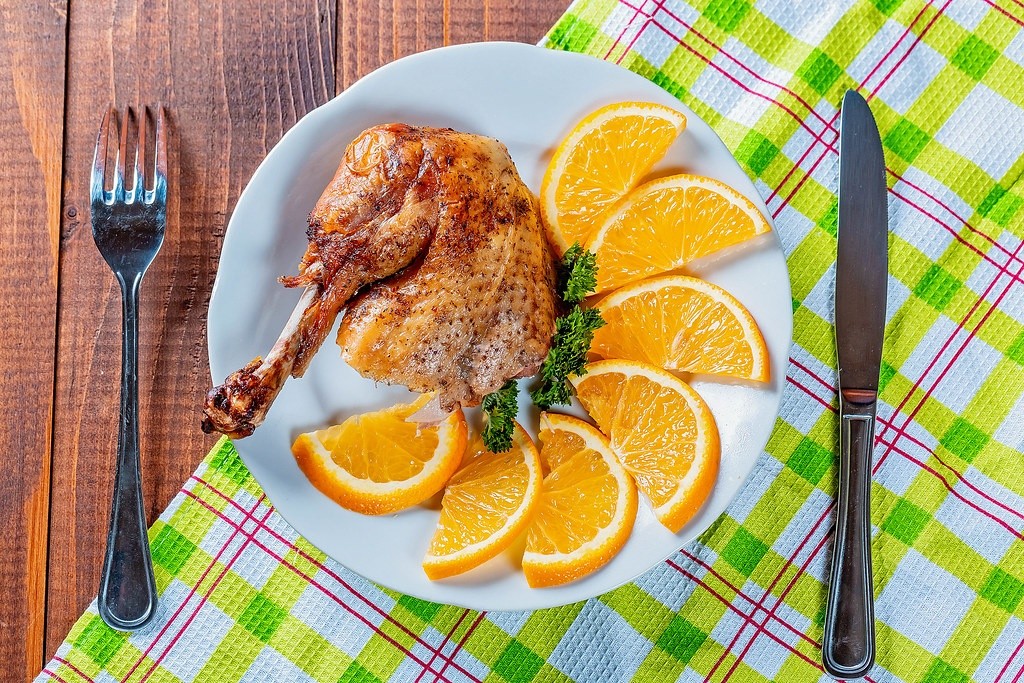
84, 100, 168, 631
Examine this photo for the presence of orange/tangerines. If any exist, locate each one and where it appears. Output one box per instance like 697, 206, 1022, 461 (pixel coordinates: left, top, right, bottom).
290, 99, 776, 587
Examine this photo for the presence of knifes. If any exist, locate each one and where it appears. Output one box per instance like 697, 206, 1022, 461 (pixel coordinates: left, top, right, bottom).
821, 88, 893, 679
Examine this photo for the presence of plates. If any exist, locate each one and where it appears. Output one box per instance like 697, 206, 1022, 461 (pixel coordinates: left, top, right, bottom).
204, 41, 792, 615
